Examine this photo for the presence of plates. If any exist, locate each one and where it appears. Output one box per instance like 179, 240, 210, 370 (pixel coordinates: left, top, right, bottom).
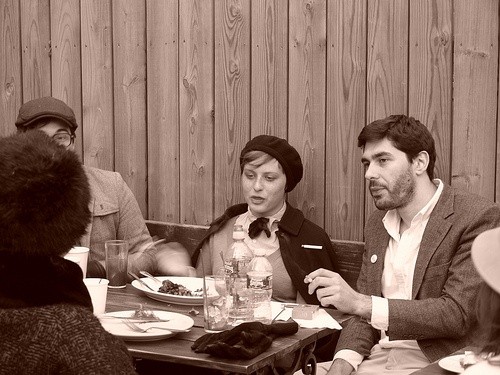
132, 277, 220, 306
437, 354, 486, 374
96, 310, 195, 342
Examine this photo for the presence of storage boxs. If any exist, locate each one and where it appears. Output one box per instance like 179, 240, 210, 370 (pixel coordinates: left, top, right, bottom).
292, 304, 319, 319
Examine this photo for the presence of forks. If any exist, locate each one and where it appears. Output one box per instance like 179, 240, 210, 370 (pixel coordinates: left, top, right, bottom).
122, 319, 191, 333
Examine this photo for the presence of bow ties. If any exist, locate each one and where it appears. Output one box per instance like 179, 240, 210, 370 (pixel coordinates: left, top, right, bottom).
247, 216, 272, 238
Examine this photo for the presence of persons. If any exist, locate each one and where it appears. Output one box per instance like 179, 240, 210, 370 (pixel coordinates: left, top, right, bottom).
0, 130, 136, 375
294, 115, 500, 375
154, 134, 340, 345
16, 97, 152, 283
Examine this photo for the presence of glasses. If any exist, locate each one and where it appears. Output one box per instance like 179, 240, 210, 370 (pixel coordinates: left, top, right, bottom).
50, 133, 75, 148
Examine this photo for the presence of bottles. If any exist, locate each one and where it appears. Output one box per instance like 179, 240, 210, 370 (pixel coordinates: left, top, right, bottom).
248, 248, 273, 324
223, 231, 255, 326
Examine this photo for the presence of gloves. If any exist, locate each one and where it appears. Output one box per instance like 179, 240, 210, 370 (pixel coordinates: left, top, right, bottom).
190, 318, 299, 359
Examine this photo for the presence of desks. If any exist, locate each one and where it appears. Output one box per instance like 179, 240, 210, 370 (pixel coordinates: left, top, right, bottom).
105, 283, 357, 375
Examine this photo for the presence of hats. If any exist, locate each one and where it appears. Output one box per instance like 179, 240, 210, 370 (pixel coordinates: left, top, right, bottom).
15, 96, 78, 131
471, 227, 500, 294
239, 135, 303, 192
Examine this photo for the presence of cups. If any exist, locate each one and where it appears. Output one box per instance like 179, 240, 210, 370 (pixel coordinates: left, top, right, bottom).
82, 278, 108, 314
63, 247, 89, 278
105, 239, 129, 288
203, 275, 231, 333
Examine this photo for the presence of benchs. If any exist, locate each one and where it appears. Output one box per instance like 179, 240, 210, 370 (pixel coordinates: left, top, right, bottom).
145, 220, 366, 363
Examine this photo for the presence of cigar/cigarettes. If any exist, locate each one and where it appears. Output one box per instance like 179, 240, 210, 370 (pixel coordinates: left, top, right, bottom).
306, 275, 312, 282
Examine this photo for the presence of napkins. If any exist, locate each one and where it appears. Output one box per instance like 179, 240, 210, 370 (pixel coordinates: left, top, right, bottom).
233, 301, 342, 331
